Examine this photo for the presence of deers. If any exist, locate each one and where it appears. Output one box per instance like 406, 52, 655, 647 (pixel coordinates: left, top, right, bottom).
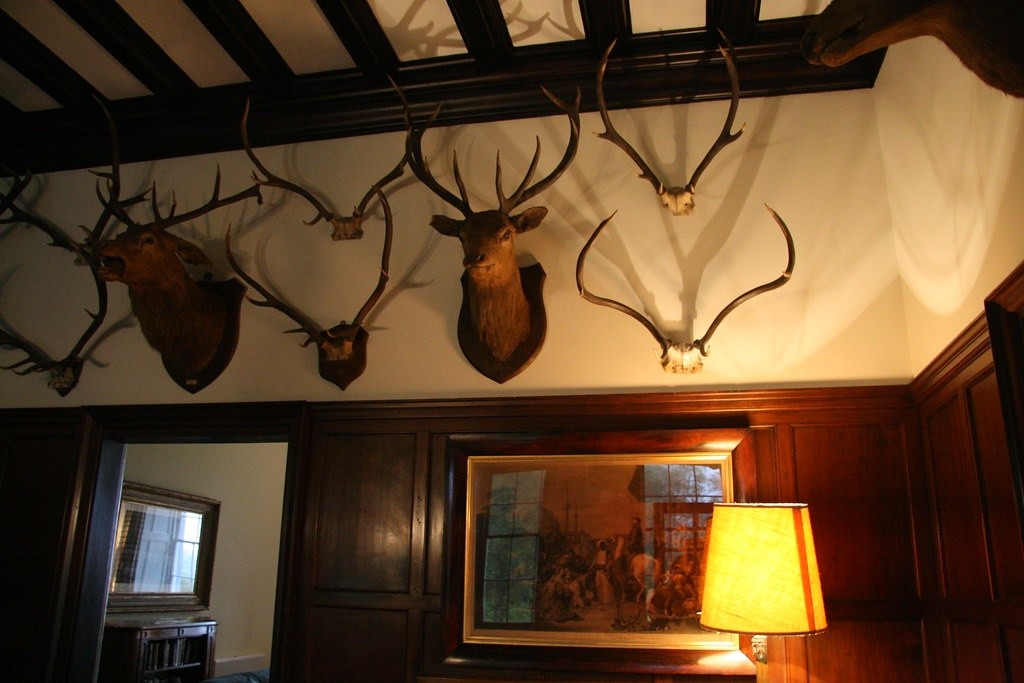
91, 175, 259, 392
405, 84, 586, 381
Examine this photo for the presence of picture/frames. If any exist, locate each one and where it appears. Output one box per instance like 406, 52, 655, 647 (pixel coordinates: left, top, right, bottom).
438, 427, 759, 677
104, 479, 222, 615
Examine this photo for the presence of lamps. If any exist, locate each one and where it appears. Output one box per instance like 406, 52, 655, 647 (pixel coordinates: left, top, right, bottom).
699, 502, 828, 664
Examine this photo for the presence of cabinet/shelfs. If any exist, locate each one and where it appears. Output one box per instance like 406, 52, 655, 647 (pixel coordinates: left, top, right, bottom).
96, 618, 220, 683
